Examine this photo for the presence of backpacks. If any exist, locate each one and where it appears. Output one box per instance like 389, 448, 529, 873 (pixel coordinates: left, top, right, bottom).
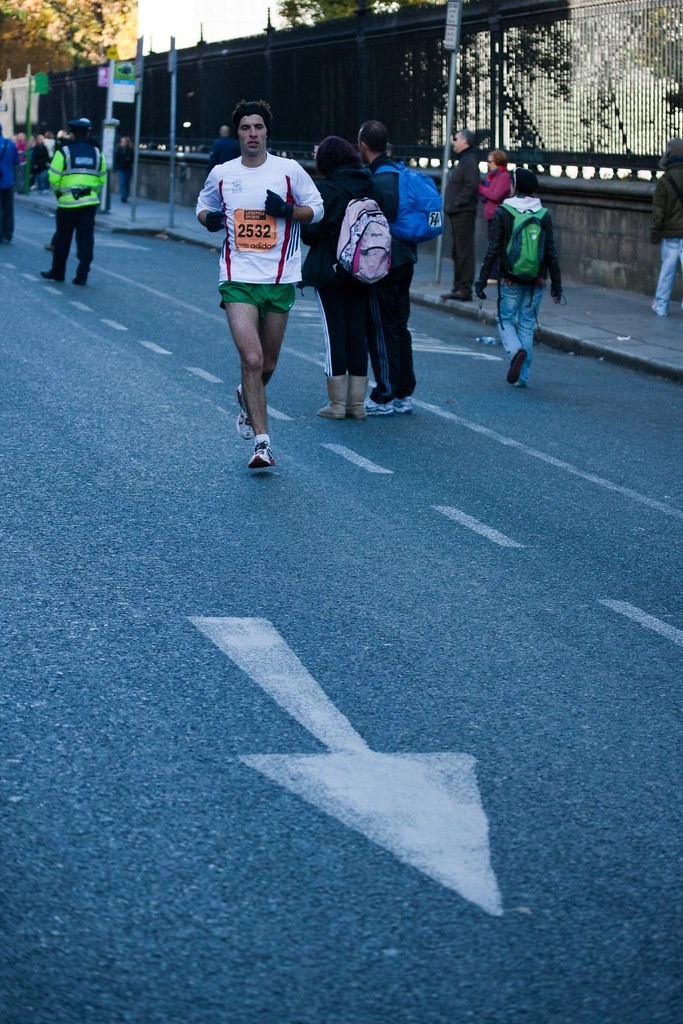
375, 161, 444, 243
500, 203, 550, 283
326, 181, 393, 284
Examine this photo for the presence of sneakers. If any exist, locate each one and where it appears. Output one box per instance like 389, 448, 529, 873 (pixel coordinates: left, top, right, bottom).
393, 397, 414, 413
236, 384, 255, 439
364, 399, 394, 416
247, 441, 275, 468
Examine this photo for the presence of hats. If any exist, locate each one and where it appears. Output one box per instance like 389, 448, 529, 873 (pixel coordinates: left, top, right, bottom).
68, 120, 91, 134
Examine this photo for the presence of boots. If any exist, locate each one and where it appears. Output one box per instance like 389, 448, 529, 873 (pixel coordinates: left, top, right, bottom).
317, 375, 348, 418
346, 373, 368, 418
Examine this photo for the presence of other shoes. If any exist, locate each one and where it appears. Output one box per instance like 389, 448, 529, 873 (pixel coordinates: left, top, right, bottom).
652, 302, 667, 316
442, 289, 473, 302
44, 243, 54, 250
73, 279, 85, 285
515, 381, 524, 386
42, 271, 64, 282
487, 279, 497, 284
507, 350, 526, 384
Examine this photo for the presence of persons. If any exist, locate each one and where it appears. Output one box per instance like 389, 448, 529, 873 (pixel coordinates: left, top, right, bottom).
475, 167, 562, 388
0, 123, 20, 245
39, 118, 108, 285
357, 122, 419, 416
196, 99, 324, 469
11, 130, 72, 195
296, 136, 377, 421
478, 149, 511, 286
647, 137, 683, 318
208, 125, 242, 165
440, 130, 479, 301
113, 136, 135, 203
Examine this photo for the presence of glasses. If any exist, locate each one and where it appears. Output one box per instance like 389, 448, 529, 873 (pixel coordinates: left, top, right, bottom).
454, 137, 463, 141
488, 160, 495, 164
514, 167, 531, 187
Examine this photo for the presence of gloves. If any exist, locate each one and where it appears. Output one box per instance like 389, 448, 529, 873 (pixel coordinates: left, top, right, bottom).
206, 210, 226, 232
475, 281, 487, 300
550, 281, 562, 302
264, 189, 294, 219
